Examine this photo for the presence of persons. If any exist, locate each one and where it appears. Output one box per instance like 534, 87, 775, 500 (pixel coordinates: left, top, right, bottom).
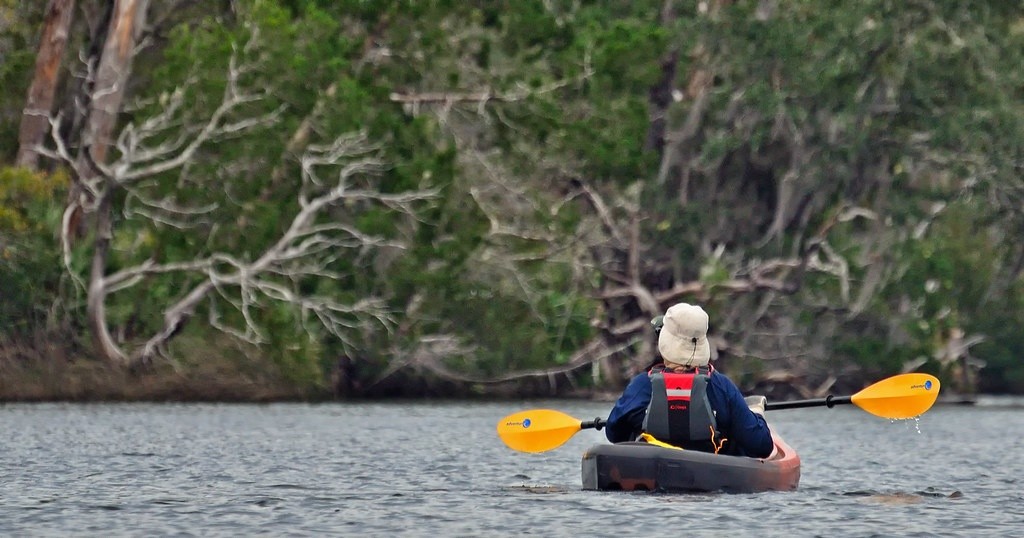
606, 303, 776, 460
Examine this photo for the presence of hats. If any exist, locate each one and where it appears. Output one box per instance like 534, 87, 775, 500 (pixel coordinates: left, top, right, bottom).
650, 302, 710, 367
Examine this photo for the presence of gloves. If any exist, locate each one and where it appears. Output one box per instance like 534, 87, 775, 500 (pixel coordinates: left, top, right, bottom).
744, 395, 767, 419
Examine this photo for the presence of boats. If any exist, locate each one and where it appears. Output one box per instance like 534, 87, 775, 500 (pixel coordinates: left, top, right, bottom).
581, 430, 800, 493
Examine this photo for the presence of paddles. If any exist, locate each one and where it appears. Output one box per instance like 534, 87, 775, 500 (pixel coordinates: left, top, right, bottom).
498, 373, 942, 450
